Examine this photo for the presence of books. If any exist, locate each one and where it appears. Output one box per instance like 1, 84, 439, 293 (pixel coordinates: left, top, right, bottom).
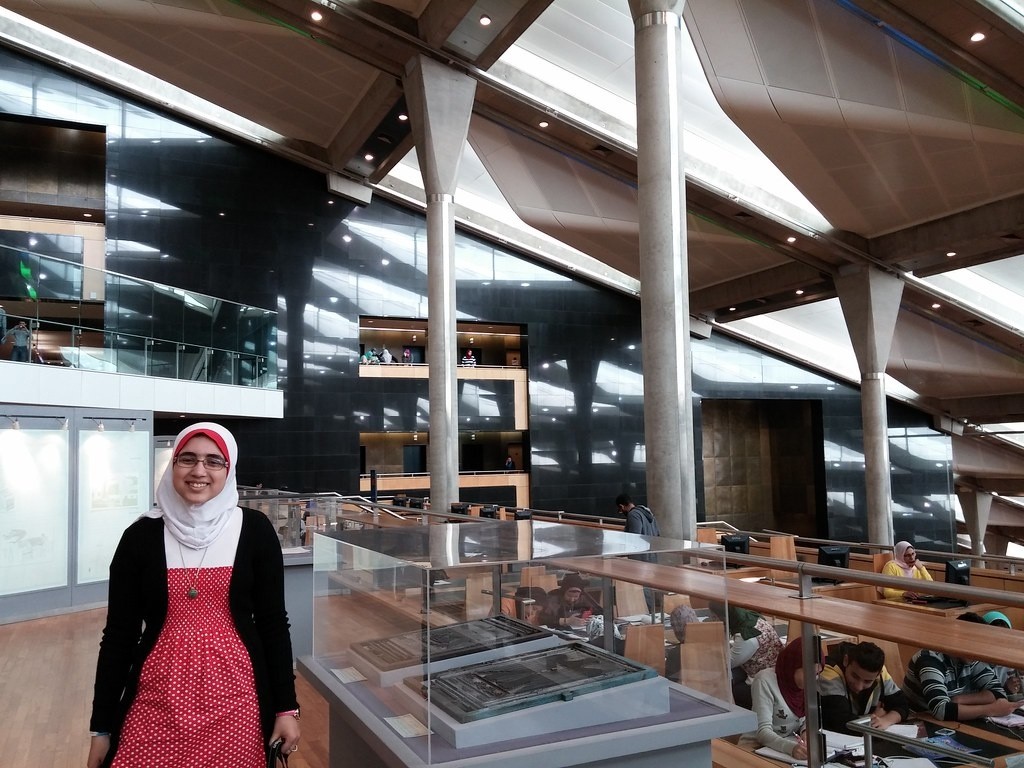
754, 728, 865, 766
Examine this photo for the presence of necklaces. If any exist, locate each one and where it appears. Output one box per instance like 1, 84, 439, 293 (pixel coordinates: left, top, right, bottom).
177, 543, 208, 598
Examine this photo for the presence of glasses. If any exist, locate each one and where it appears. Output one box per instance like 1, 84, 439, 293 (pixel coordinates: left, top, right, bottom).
174, 455, 228, 471
903, 553, 917, 556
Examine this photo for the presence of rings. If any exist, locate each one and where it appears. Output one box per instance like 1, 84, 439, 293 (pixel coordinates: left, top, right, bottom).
1015, 687, 1018, 690
291, 745, 299, 751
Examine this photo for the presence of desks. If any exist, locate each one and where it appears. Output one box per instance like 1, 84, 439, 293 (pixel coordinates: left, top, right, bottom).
557, 563, 1024, 768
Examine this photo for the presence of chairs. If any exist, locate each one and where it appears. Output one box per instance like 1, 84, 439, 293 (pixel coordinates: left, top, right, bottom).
857, 634, 905, 689
873, 552, 893, 599
625, 624, 665, 678
685, 622, 724, 642
519, 566, 557, 592
614, 579, 648, 618
770, 536, 797, 579
698, 528, 717, 544
680, 642, 731, 704
786, 618, 820, 642
664, 594, 691, 612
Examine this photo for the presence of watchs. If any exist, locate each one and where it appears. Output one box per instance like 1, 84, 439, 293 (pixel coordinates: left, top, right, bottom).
276, 708, 301, 721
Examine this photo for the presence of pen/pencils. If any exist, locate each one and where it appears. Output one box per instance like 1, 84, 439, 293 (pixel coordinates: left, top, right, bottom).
793, 731, 804, 745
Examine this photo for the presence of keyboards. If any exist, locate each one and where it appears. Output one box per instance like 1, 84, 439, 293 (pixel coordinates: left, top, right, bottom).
917, 596, 948, 603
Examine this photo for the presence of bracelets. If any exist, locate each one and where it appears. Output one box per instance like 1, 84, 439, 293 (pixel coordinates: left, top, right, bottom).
90, 731, 109, 737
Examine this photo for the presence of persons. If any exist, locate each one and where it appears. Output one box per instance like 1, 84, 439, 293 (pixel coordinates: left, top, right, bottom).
876, 541, 933, 600
736, 634, 910, 768
461, 350, 476, 368
617, 494, 660, 610
361, 347, 398, 366
292, 511, 310, 546
7, 321, 30, 362
904, 611, 1024, 721
666, 601, 784, 711
402, 349, 414, 366
87, 422, 301, 768
537, 574, 623, 654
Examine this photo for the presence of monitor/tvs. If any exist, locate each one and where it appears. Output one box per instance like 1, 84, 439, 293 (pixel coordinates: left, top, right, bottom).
720, 534, 750, 568
514, 511, 532, 520
451, 504, 469, 515
393, 497, 406, 508
480, 507, 496, 519
817, 547, 849, 585
946, 561, 971, 602
410, 498, 424, 509
